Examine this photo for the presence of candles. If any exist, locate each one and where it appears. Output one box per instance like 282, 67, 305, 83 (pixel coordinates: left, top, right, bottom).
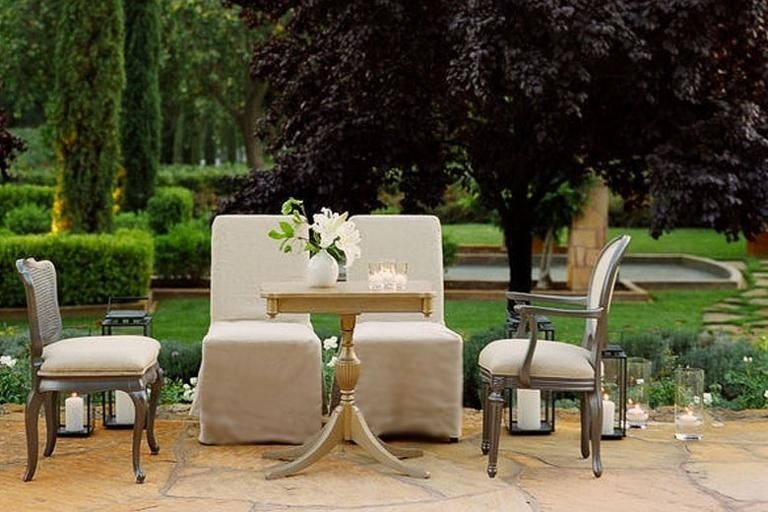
517, 388, 541, 430
114, 389, 136, 424
679, 409, 697, 434
601, 393, 615, 435
628, 403, 644, 421
64, 393, 84, 432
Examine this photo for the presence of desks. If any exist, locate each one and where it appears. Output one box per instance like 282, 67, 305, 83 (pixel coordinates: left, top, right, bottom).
504, 315, 554, 341
259, 280, 439, 480
101, 297, 153, 337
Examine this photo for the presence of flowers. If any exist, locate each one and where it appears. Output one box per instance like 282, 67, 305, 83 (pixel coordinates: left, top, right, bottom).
267, 196, 363, 260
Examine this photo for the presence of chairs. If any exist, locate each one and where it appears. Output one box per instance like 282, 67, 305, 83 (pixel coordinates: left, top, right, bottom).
342, 213, 465, 448
14, 257, 164, 486
475, 232, 634, 479
198, 214, 326, 446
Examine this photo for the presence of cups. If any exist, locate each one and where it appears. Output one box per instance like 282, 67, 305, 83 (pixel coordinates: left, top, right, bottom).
627, 358, 705, 442
368, 260, 409, 291
